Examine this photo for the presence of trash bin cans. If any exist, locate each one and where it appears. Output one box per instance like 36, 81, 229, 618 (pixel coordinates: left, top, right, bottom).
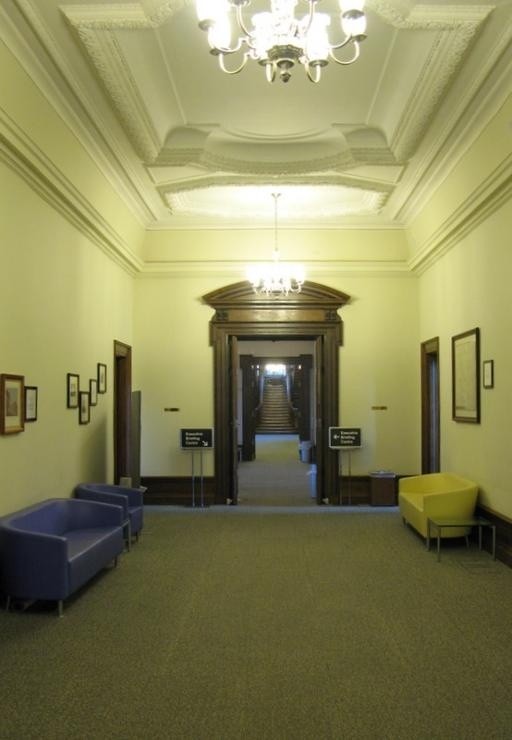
307, 464, 318, 498
302, 441, 312, 462
120, 472, 148, 493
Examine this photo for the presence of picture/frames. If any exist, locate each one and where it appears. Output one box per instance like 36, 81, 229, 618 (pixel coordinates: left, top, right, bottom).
96, 362, 108, 395
448, 327, 483, 425
77, 390, 92, 426
480, 358, 496, 390
22, 383, 39, 424
88, 377, 100, 408
0, 372, 28, 435
65, 372, 81, 409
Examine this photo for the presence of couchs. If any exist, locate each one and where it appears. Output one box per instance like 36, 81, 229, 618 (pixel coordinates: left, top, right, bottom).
1, 493, 123, 618
395, 471, 481, 554
73, 483, 146, 545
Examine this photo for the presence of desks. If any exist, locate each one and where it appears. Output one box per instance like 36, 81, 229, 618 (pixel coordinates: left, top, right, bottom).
424, 514, 501, 564
121, 516, 135, 553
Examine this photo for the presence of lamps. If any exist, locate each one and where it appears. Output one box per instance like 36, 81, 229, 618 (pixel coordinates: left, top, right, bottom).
189, 1, 372, 87
240, 191, 312, 298
